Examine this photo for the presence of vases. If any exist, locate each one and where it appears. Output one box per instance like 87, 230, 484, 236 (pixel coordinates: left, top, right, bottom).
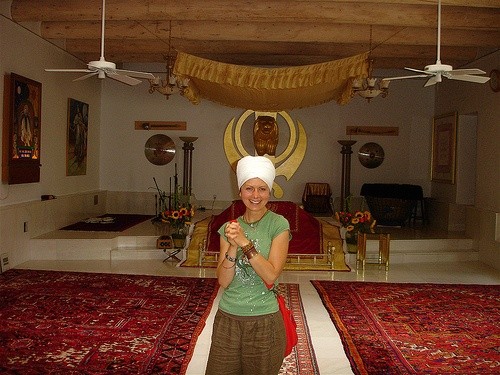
173, 235, 185, 248
346, 233, 359, 252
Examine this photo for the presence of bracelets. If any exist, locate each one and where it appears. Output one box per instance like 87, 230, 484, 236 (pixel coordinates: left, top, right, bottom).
222, 252, 237, 269
243, 243, 258, 260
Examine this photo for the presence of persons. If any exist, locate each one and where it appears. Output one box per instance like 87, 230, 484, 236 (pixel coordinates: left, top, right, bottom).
205, 156, 292, 375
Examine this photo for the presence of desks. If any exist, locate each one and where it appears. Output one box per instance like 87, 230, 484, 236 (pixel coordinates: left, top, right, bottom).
357, 224, 402, 269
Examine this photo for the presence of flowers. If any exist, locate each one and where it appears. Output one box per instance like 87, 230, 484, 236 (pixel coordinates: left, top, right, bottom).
337, 209, 375, 239
161, 202, 191, 236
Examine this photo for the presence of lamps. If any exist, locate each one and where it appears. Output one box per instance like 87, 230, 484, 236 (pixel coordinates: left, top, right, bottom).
181, 136, 197, 194
353, 27, 391, 102
338, 137, 357, 199
149, 20, 186, 102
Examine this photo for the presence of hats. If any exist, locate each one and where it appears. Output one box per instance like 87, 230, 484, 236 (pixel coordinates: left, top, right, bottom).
236, 156, 276, 192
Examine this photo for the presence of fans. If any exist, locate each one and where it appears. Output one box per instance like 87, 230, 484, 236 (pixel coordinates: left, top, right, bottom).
44, 0, 156, 87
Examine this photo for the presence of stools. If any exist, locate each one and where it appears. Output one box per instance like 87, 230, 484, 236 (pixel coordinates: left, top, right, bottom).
410, 195, 428, 224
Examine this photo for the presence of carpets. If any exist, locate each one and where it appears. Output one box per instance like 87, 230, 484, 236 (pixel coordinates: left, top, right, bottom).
61, 213, 156, 231
310, 276, 500, 374
0, 268, 219, 375
276, 283, 317, 374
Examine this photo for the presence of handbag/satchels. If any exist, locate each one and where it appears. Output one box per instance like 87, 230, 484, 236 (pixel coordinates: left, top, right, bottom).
274, 294, 298, 356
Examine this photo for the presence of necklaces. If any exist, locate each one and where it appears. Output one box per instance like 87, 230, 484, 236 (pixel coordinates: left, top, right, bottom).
244, 209, 268, 227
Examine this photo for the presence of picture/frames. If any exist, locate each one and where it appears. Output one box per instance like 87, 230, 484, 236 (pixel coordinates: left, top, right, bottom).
9, 72, 41, 183
66, 97, 88, 176
